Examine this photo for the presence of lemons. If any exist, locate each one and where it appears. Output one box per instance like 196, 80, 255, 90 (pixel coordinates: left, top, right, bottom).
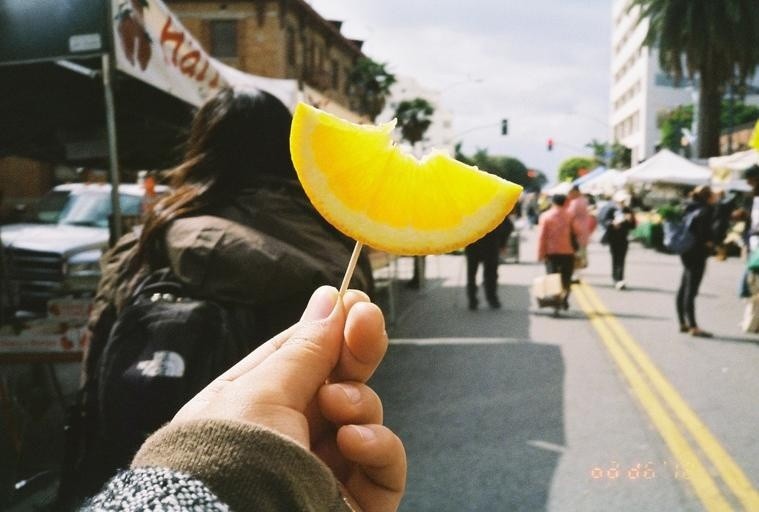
289, 100, 524, 256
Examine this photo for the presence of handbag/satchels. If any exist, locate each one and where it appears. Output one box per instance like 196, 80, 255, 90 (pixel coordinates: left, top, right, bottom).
532, 272, 567, 307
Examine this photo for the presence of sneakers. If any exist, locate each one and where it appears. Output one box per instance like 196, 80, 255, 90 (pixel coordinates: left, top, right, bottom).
681, 328, 714, 338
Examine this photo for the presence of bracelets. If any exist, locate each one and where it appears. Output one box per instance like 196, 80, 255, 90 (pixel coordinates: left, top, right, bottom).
713, 243, 720, 254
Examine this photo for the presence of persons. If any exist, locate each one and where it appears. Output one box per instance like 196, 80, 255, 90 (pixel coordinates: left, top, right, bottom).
534, 193, 579, 312
462, 216, 516, 310
561, 184, 589, 268
730, 162, 759, 335
73, 284, 409, 512
597, 191, 637, 292
73, 86, 377, 484
676, 184, 730, 340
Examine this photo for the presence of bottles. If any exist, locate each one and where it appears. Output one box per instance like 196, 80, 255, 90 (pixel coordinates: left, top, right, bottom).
137, 171, 156, 217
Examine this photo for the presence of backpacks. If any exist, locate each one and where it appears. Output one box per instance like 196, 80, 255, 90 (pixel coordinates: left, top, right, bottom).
52, 264, 241, 512
659, 208, 713, 255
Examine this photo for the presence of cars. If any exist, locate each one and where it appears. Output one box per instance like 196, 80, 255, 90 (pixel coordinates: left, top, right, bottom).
0, 181, 170, 320
